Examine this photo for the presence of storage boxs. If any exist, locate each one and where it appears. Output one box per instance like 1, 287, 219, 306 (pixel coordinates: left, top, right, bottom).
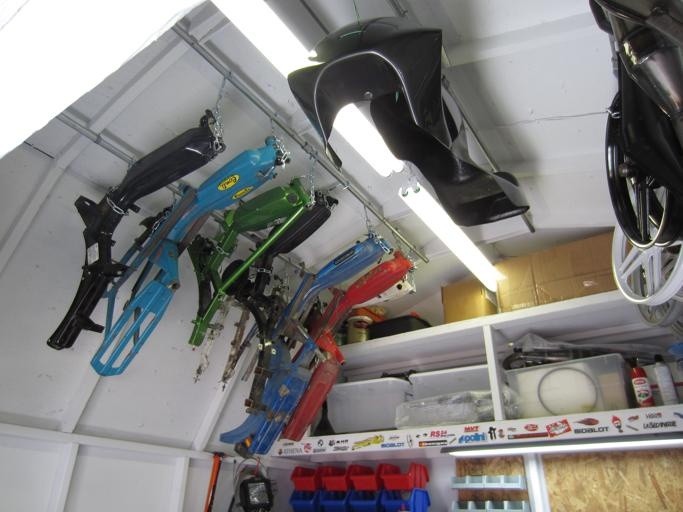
323, 377, 410, 434
410, 365, 489, 399
365, 315, 430, 340
503, 353, 630, 419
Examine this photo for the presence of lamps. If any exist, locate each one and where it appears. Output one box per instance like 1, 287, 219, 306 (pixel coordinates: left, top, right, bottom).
397, 178, 505, 293
436, 430, 683, 458
210, 0, 406, 177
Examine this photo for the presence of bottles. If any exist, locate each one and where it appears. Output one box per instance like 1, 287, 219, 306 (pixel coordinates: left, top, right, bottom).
628, 357, 655, 407
653, 354, 679, 405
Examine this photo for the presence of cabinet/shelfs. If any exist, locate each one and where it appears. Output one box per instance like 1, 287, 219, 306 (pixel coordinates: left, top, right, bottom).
260, 282, 682, 461
